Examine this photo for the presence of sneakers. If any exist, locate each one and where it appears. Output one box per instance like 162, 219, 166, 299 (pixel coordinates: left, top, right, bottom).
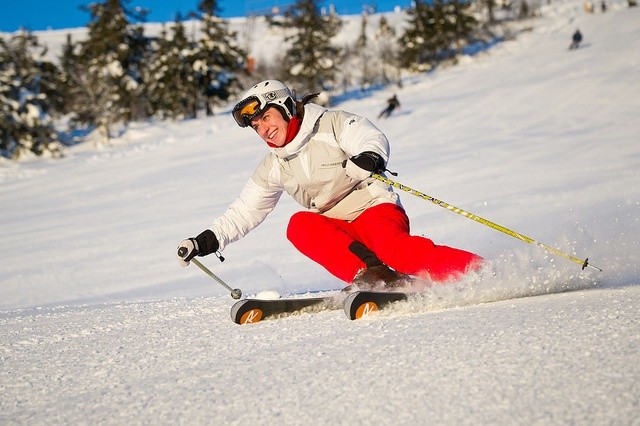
351, 265, 415, 291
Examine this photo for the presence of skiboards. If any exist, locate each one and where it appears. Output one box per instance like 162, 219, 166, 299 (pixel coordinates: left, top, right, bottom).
229, 290, 444, 325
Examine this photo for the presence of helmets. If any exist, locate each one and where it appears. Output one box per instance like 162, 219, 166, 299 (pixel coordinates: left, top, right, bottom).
232, 80, 296, 123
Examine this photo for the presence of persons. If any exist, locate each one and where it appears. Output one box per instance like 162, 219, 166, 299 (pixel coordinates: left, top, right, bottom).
567, 28, 584, 51
176, 79, 491, 291
375, 94, 401, 121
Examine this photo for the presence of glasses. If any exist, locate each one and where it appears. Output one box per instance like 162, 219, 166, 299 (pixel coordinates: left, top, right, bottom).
232, 87, 292, 127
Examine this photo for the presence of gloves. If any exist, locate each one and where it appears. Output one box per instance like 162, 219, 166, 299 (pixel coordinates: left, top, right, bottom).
346, 151, 397, 186
176, 230, 225, 262
